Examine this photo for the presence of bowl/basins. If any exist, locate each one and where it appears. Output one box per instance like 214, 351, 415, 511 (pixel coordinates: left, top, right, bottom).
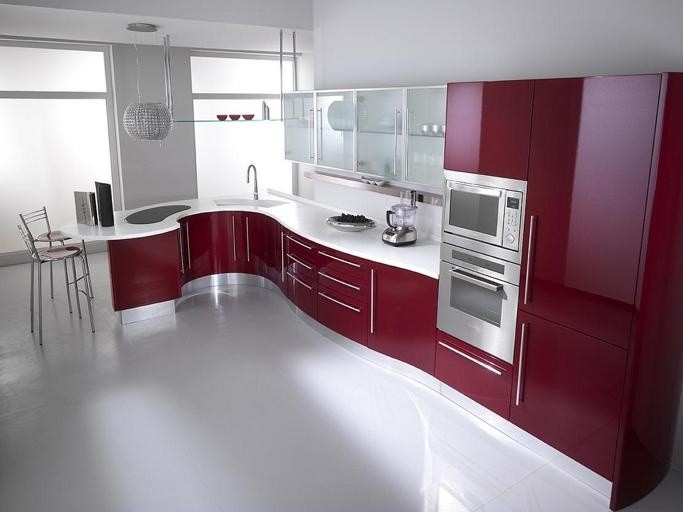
421, 123, 445, 136
243, 114, 254, 121
216, 115, 228, 121
327, 215, 376, 233
362, 178, 385, 187
229, 115, 241, 121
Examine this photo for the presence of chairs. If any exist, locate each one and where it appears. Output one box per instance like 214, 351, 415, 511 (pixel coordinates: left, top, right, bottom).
19, 206, 94, 313
18, 226, 95, 345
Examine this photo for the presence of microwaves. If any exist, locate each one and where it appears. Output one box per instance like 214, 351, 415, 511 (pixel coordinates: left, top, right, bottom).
443, 180, 523, 252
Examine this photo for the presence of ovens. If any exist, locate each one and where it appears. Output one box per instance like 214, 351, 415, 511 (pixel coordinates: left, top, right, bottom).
434, 260, 519, 366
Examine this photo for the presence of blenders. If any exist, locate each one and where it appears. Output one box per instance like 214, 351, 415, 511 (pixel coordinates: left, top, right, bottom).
380, 188, 418, 248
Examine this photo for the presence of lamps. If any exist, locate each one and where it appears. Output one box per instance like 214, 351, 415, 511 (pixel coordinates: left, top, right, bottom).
123, 24, 173, 141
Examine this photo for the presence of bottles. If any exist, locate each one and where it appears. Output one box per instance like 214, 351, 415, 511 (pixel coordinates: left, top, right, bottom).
262, 100, 267, 121
265, 104, 270, 121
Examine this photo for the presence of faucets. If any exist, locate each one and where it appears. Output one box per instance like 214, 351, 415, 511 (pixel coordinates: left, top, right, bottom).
248, 165, 258, 200
